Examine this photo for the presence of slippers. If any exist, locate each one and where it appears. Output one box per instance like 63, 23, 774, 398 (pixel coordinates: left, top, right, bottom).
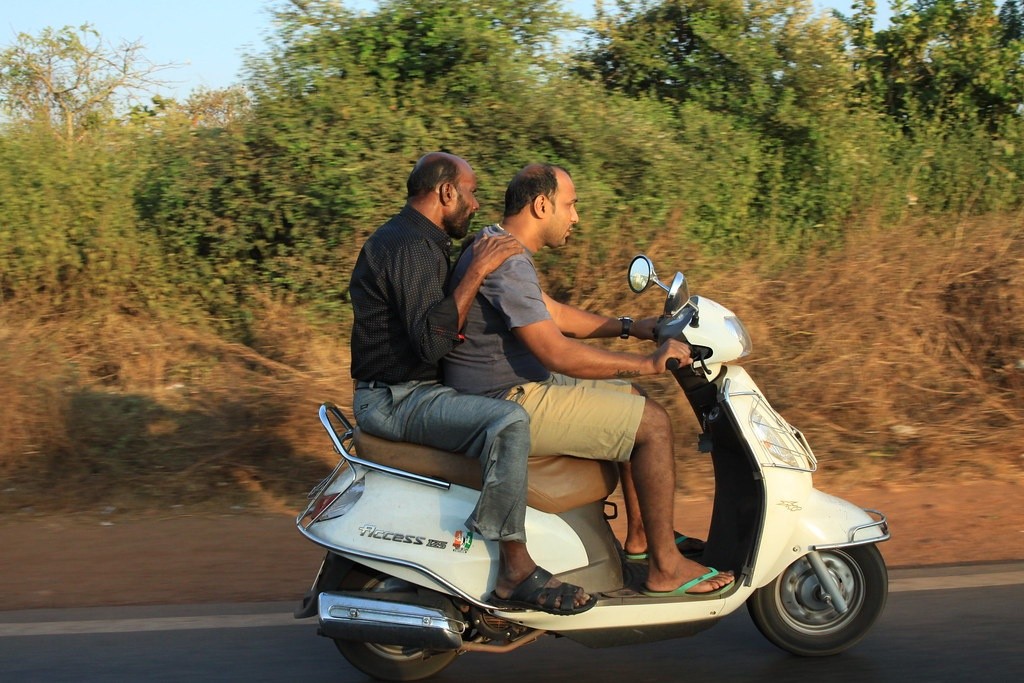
624, 534, 708, 559
638, 565, 737, 598
490, 565, 599, 615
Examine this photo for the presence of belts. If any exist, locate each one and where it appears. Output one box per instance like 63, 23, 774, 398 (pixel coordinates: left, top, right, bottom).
354, 379, 399, 390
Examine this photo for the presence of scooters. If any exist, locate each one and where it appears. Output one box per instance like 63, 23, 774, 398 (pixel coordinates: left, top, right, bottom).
297, 253, 893, 683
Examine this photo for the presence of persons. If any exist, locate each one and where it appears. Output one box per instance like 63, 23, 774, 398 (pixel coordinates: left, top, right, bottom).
448, 164, 736, 596
351, 152, 597, 616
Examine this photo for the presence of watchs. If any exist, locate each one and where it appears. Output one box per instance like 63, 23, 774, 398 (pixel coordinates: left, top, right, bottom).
617, 315, 634, 339
